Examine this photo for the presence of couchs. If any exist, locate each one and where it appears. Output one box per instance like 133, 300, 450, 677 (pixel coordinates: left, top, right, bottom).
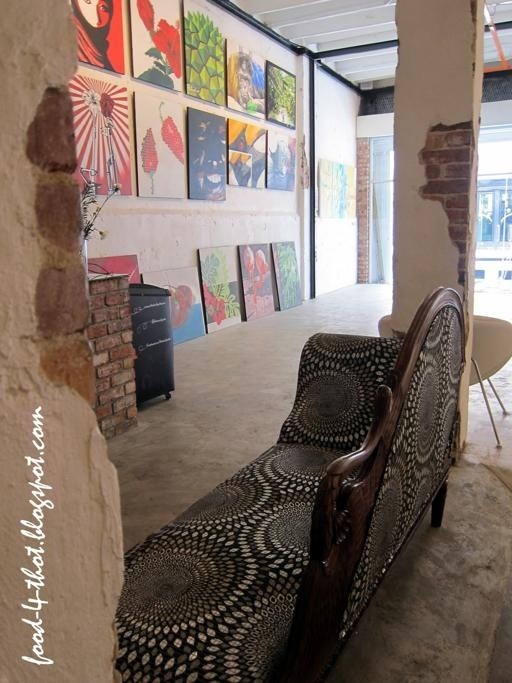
120, 285, 468, 683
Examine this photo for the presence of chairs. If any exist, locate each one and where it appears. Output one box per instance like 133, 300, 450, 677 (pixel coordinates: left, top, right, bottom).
377, 311, 512, 448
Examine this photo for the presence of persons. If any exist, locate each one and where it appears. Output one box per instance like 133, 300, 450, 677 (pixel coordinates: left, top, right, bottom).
71, 0, 121, 72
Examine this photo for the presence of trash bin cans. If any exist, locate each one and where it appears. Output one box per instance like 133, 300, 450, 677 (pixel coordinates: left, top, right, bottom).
129, 284, 175, 411
